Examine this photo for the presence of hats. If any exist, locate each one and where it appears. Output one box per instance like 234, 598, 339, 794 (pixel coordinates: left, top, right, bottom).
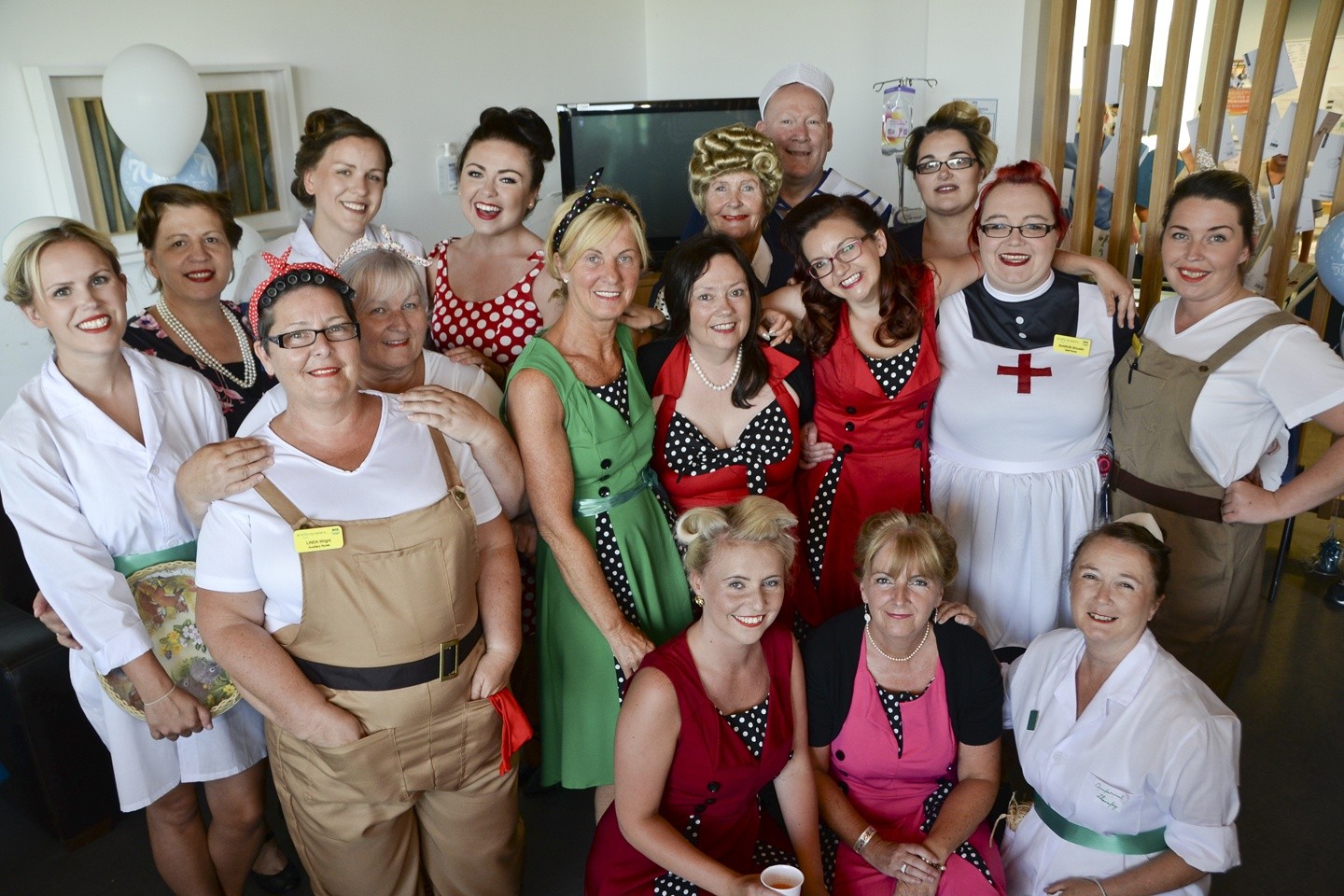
758, 63, 834, 122
1, 216, 68, 266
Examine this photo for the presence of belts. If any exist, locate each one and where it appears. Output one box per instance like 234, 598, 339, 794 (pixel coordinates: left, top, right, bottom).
292, 619, 482, 691
1109, 461, 1222, 524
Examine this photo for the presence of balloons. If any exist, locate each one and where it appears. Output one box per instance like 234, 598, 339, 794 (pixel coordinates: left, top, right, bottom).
100, 45, 209, 177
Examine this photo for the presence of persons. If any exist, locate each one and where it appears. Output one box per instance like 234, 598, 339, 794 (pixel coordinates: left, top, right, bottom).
0, 56, 1344, 896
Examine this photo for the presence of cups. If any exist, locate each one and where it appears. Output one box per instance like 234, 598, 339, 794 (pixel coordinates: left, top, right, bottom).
760, 864, 805, 896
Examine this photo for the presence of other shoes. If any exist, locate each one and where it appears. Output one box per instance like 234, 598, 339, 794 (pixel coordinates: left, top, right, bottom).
1326, 576, 1344, 609
251, 858, 301, 894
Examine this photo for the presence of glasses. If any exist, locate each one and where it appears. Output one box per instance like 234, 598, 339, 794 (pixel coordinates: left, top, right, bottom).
806, 231, 874, 280
265, 321, 362, 348
914, 157, 977, 174
978, 223, 1059, 238
